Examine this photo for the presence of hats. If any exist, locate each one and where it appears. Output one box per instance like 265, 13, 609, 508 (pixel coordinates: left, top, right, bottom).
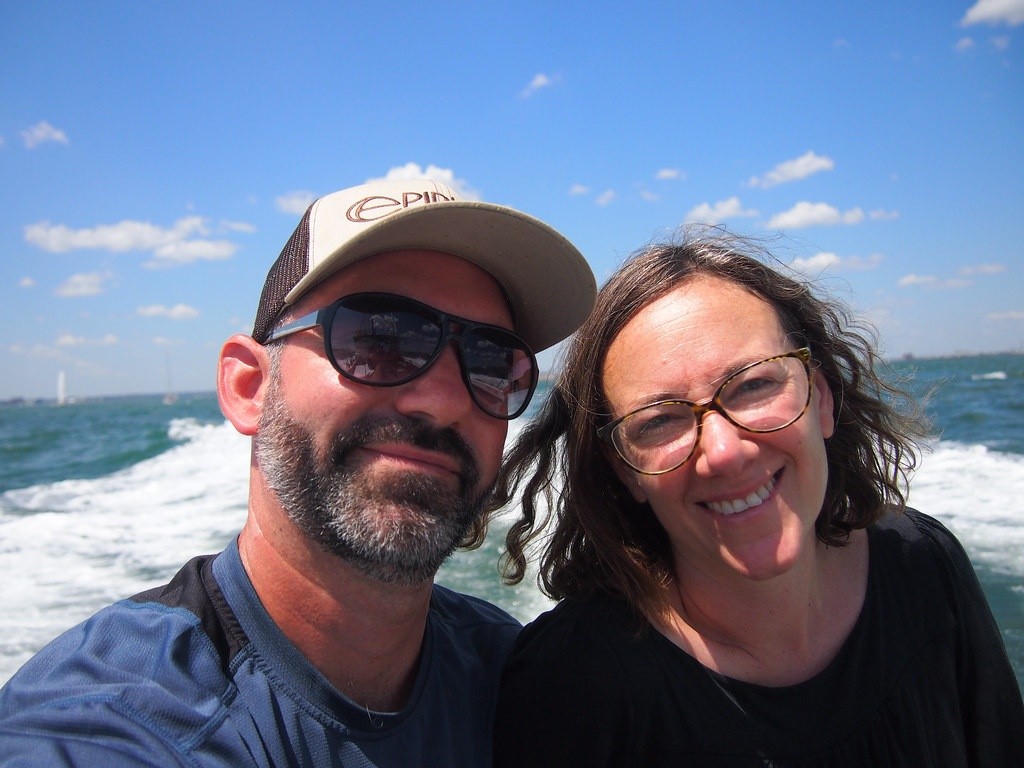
252, 179, 598, 345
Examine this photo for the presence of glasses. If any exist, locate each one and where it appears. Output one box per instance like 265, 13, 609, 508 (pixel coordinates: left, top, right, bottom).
597, 343, 812, 475
262, 292, 539, 420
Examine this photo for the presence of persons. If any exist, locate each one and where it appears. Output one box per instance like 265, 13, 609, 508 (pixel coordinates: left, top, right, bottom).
0, 174, 581, 768
489, 232, 1024, 768
